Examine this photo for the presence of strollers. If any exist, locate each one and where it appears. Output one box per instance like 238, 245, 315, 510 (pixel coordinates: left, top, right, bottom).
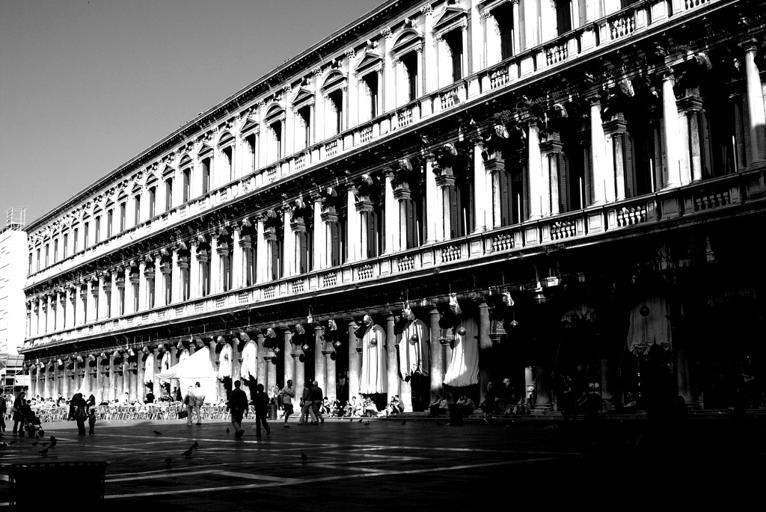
17, 404, 45, 437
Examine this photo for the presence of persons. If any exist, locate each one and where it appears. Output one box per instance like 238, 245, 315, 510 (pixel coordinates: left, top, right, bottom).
0, 381, 205, 438
226, 379, 403, 439
556, 374, 602, 419
428, 377, 517, 425
